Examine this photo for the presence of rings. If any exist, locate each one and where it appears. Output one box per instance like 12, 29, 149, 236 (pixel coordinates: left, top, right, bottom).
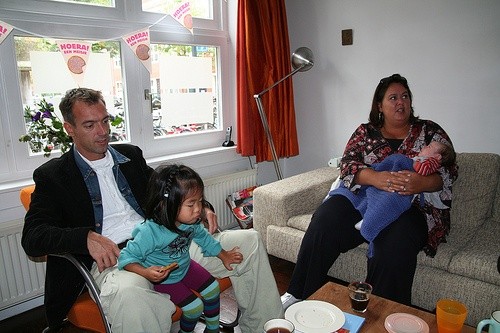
388, 182, 392, 186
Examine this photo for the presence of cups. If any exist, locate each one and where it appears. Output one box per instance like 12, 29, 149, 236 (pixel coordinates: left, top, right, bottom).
476, 310, 500, 333
436, 299, 467, 333
263, 318, 295, 333
347, 281, 372, 313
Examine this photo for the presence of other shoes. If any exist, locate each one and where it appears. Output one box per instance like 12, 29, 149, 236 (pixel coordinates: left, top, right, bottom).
280, 292, 302, 310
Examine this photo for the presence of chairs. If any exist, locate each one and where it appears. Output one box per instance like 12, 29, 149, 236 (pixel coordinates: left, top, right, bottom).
19, 185, 242, 333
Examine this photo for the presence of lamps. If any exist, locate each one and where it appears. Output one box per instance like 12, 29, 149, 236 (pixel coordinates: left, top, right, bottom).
254, 47, 315, 181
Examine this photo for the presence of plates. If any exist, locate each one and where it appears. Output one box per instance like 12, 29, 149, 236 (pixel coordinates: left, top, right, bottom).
384, 312, 430, 333
285, 300, 345, 333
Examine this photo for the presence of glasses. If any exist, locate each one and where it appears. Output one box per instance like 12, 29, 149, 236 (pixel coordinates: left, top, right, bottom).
379, 74, 400, 88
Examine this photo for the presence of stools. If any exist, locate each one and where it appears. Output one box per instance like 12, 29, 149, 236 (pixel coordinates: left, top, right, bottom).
226, 184, 258, 229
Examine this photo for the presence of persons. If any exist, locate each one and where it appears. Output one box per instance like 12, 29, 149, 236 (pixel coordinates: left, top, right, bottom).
354, 140, 456, 231
118, 165, 244, 333
22, 87, 285, 333
281, 74, 459, 311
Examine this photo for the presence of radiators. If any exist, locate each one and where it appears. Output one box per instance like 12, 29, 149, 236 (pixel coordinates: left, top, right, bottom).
0, 163, 260, 311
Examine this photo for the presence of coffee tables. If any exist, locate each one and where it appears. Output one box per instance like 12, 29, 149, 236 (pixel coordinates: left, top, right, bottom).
306, 282, 487, 333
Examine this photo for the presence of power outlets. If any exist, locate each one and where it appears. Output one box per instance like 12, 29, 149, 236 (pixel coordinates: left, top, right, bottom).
342, 29, 353, 45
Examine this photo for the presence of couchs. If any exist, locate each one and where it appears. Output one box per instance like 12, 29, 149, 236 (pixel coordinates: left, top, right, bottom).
252, 152, 500, 330
